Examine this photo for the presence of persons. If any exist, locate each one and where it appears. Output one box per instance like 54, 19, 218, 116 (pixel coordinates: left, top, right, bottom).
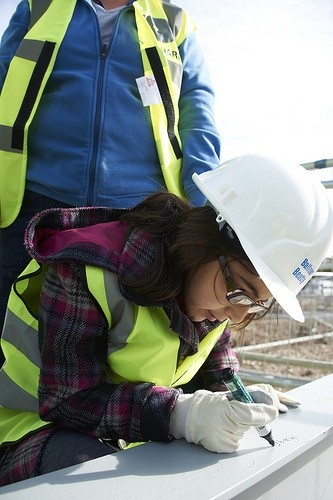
0, 153, 333, 487
0, 0, 222, 333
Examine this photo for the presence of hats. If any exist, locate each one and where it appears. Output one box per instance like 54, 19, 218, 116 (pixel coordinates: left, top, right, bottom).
189, 155, 333, 323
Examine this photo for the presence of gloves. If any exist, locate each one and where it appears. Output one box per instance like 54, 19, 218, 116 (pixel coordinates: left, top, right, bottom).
171, 389, 279, 455
242, 383, 306, 413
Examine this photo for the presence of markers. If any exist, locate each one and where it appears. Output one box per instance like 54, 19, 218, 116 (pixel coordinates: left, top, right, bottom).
220, 368, 275, 447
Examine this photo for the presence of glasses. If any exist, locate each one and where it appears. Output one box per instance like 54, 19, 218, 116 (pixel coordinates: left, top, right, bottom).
213, 252, 273, 314
